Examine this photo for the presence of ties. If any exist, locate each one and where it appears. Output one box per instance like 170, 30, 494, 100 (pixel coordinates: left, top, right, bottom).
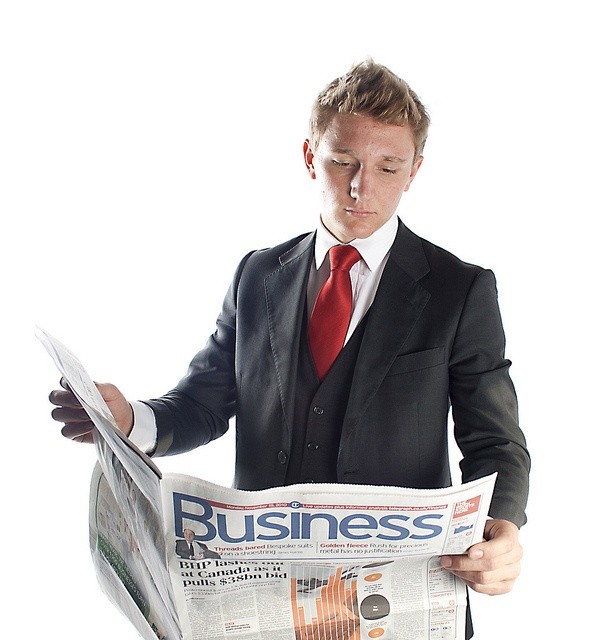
308, 244, 362, 382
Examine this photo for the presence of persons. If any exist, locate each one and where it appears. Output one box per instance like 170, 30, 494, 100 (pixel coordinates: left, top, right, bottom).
49, 58, 531, 640
